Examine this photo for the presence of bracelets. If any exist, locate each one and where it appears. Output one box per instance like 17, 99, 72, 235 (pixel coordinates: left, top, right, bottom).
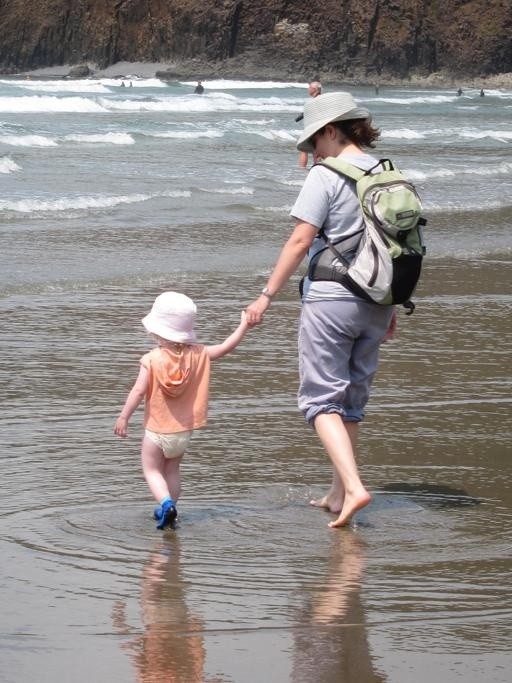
261, 286, 273, 299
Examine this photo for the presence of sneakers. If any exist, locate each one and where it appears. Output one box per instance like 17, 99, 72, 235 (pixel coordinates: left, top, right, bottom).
154, 501, 178, 529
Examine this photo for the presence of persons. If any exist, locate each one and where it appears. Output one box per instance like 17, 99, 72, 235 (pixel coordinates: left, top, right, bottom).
194, 80, 205, 95
297, 80, 322, 168
245, 90, 399, 529
112, 289, 252, 528
110, 529, 209, 682
272, 528, 387, 683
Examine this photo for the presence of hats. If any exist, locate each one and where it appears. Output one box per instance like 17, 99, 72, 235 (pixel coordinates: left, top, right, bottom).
141, 292, 198, 345
294, 91, 371, 153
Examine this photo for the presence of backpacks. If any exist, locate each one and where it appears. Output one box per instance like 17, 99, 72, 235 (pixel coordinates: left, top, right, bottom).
317, 155, 427, 316
